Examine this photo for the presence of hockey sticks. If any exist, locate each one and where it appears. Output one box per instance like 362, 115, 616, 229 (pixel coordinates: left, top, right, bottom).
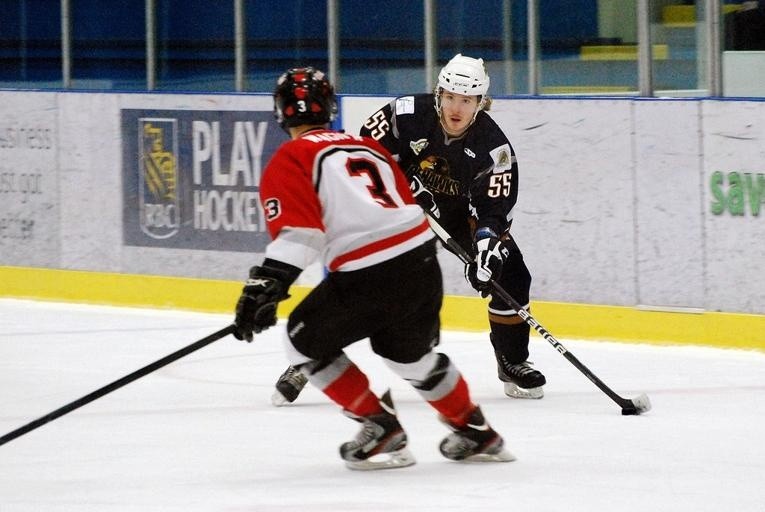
424, 211, 653, 413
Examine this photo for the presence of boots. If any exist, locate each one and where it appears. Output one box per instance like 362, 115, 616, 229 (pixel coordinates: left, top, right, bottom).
495, 351, 546, 389
340, 388, 407, 462
276, 365, 308, 402
439, 405, 503, 460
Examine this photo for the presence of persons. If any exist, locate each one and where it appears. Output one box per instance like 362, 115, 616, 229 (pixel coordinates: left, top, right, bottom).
234, 65, 505, 465
275, 54, 547, 402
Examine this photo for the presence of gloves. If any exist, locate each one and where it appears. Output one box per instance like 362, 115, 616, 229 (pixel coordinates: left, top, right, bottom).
232, 266, 291, 342
408, 175, 441, 221
465, 238, 509, 297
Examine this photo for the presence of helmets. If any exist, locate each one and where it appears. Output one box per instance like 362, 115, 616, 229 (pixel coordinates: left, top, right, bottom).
437, 54, 490, 96
274, 67, 338, 128
